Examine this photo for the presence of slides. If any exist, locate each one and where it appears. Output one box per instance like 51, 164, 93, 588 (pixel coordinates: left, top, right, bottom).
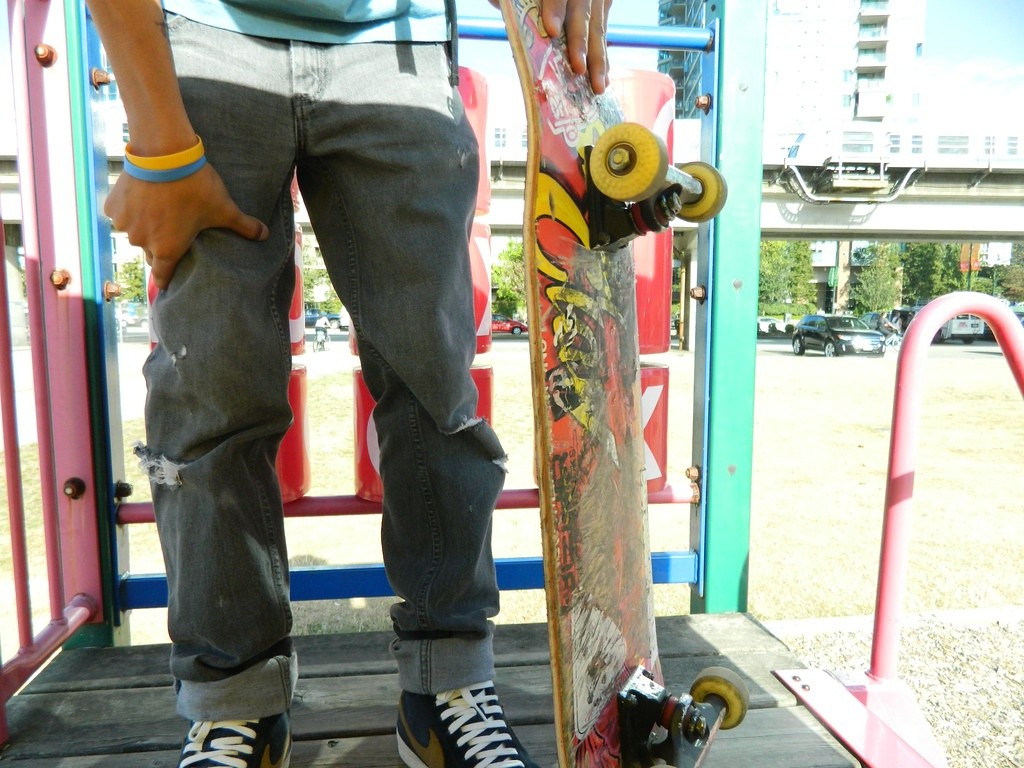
498, 0, 755, 768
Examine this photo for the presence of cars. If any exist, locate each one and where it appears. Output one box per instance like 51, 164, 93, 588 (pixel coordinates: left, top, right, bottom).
859, 312, 891, 331
304, 306, 343, 330
491, 312, 529, 336
758, 317, 796, 335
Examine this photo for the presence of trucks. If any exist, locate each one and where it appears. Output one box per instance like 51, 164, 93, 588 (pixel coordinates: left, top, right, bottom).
891, 298, 985, 345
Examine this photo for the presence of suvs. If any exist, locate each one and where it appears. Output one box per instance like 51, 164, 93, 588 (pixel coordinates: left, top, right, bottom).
791, 314, 887, 358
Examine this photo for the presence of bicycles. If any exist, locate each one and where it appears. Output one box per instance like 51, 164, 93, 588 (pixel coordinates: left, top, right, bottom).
311, 326, 333, 353
875, 326, 903, 352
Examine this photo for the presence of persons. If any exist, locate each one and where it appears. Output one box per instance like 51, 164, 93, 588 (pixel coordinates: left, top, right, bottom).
78, 1, 621, 768
315, 311, 331, 351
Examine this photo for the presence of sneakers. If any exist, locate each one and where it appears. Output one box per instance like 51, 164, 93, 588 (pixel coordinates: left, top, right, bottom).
178, 714, 294, 768
394, 681, 536, 768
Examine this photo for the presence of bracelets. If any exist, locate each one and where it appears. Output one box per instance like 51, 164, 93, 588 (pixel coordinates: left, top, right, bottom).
123, 132, 203, 169
122, 154, 208, 182
